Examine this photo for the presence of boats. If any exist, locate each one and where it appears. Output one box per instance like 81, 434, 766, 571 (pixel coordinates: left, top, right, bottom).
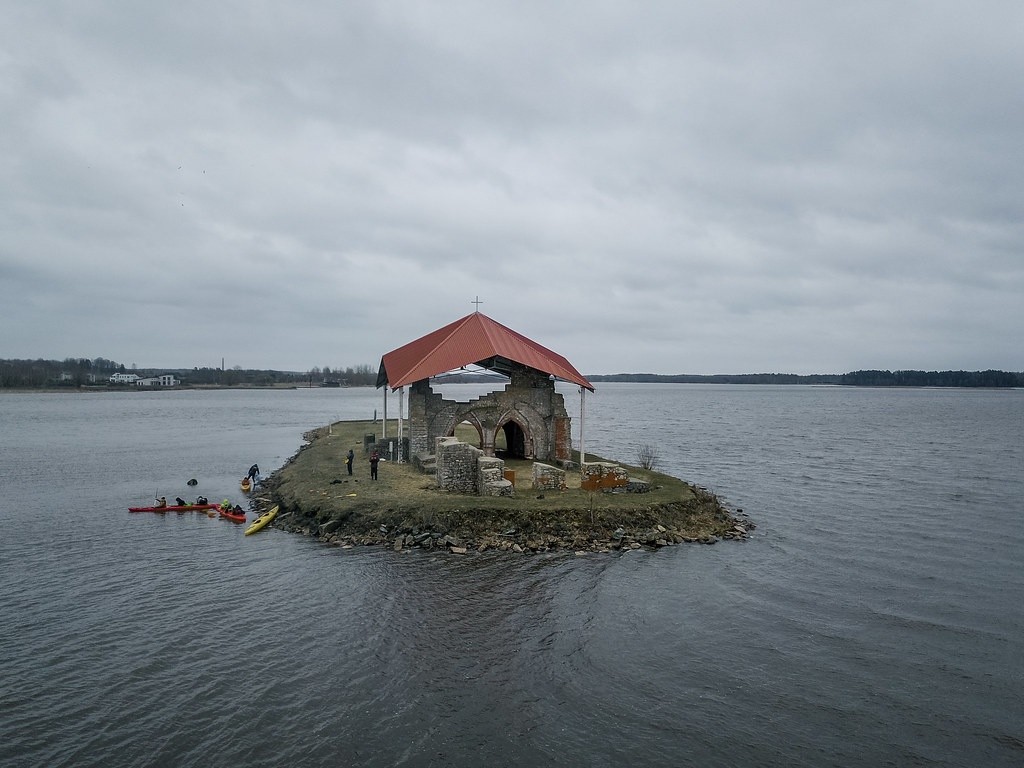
167, 503, 215, 511
216, 504, 246, 521
128, 506, 169, 511
241, 480, 251, 490
244, 506, 279, 536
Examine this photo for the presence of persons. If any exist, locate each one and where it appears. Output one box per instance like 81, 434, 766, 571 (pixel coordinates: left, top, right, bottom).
246, 464, 259, 483
369, 451, 380, 480
221, 499, 245, 515
154, 496, 166, 507
176, 497, 185, 506
345, 449, 354, 476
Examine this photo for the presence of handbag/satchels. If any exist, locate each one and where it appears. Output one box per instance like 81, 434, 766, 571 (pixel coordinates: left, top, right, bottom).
344, 459, 349, 464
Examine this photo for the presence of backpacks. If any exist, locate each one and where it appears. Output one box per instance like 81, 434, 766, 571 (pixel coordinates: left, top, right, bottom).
371, 455, 378, 462
